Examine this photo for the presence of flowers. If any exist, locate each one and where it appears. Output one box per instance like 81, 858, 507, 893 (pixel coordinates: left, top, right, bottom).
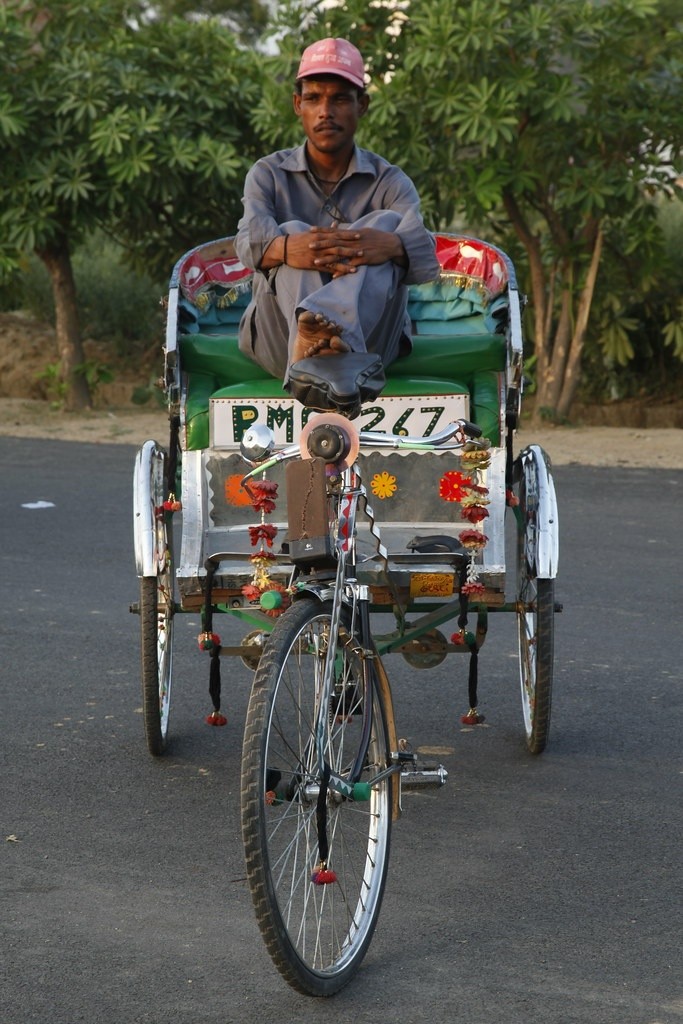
242, 468, 306, 617
440, 437, 491, 597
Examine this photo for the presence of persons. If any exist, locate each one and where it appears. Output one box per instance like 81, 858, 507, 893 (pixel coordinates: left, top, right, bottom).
233, 37, 441, 394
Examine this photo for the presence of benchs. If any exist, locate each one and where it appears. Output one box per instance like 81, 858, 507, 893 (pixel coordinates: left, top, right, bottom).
166, 232, 522, 452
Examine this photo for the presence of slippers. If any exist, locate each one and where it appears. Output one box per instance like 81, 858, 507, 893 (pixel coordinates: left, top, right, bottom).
405, 535, 477, 553
277, 532, 291, 554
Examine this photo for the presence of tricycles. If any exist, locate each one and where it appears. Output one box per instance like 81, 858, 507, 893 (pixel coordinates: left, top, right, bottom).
130, 228, 570, 998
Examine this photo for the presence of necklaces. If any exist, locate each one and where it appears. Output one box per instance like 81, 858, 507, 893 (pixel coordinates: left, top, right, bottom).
313, 172, 337, 185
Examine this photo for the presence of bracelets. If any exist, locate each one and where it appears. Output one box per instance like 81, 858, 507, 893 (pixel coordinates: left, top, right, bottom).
284, 233, 289, 264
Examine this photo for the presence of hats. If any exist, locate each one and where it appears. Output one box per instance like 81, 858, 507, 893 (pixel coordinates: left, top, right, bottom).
296, 37, 365, 89
